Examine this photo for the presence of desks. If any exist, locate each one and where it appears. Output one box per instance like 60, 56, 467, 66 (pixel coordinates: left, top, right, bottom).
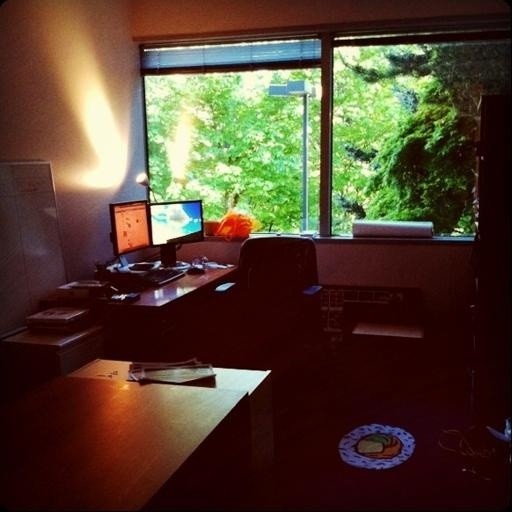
42, 261, 238, 362
0, 359, 276, 511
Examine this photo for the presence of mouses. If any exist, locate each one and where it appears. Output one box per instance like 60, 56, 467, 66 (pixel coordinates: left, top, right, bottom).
189, 265, 204, 273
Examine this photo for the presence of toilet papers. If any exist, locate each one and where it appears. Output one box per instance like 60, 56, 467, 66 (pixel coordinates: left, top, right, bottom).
353, 220, 433, 237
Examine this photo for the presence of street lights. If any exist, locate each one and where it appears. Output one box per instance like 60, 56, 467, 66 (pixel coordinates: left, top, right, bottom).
270, 79, 323, 235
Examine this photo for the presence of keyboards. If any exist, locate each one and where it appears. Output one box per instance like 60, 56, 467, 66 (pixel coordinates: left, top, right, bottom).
146, 268, 186, 287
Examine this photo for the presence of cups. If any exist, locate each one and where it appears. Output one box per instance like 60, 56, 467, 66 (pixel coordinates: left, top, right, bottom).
192, 257, 209, 269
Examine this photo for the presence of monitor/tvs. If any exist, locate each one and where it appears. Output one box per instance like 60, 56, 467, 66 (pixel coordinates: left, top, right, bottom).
109, 199, 155, 275
147, 198, 205, 270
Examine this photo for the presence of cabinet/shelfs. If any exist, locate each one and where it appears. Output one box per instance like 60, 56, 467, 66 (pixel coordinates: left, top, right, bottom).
1, 322, 106, 392
1, 160, 79, 335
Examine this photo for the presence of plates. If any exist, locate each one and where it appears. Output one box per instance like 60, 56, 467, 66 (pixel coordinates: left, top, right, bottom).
337, 423, 417, 470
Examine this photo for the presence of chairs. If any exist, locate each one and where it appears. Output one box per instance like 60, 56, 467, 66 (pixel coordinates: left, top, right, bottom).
177, 239, 320, 363
